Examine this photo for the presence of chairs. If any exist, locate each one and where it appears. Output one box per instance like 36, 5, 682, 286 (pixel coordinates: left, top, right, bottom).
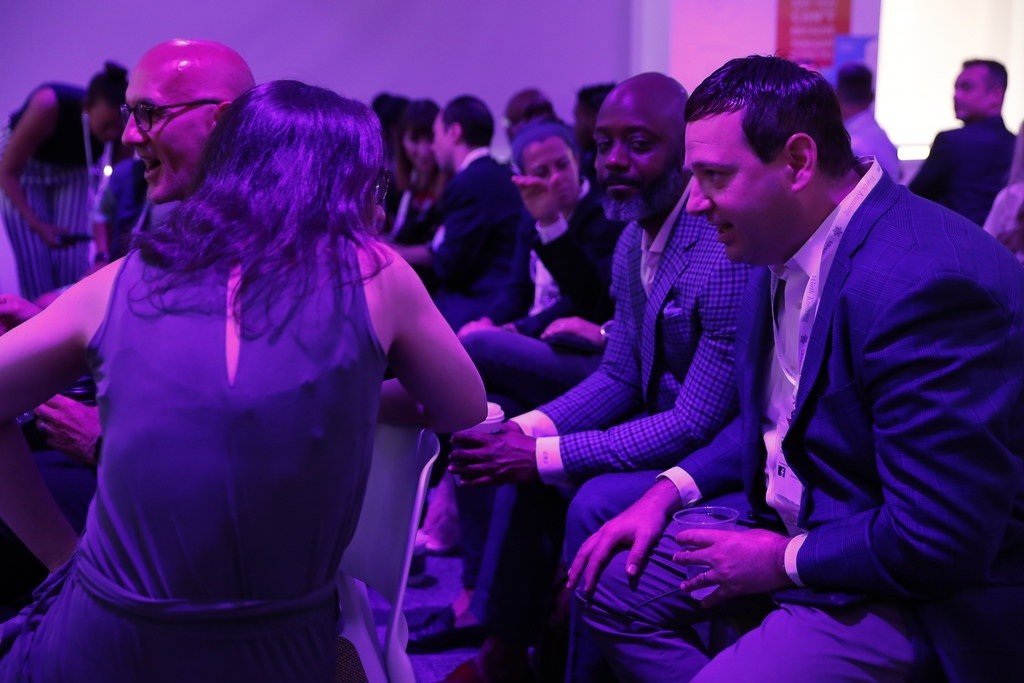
332, 420, 439, 683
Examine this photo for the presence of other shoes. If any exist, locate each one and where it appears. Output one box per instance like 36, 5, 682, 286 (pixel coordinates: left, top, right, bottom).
408, 543, 465, 576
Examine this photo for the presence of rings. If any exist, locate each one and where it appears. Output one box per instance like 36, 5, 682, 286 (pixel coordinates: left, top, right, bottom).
704, 573, 708, 585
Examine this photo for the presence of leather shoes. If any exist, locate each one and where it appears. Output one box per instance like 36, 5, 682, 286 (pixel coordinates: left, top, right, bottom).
406, 611, 490, 654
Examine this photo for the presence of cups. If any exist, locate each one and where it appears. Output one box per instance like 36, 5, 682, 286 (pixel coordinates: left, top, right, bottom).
673, 507, 739, 600
452, 400, 505, 487
55, 377, 98, 448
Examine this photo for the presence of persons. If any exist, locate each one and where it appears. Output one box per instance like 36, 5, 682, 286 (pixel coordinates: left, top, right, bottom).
0, 80, 488, 683
907, 60, 1017, 228
0, 38, 1024, 683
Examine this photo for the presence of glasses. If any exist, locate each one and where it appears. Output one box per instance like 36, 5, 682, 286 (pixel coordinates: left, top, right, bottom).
119, 99, 220, 130
